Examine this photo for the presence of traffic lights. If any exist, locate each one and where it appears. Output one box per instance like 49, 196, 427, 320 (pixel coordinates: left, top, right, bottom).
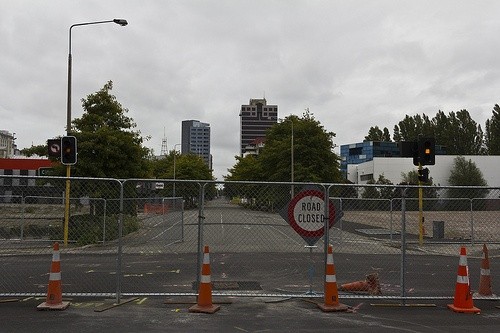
420, 137, 436, 165
418, 168, 428, 183
60, 135, 77, 164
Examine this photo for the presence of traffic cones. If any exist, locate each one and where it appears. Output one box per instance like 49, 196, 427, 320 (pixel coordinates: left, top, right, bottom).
188, 245, 222, 314
338, 274, 381, 295
477, 243, 493, 295
36, 242, 71, 310
446, 246, 481, 313
316, 244, 349, 312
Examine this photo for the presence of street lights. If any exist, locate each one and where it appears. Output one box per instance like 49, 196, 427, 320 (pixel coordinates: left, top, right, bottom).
62, 18, 128, 249
267, 115, 295, 199
172, 142, 193, 210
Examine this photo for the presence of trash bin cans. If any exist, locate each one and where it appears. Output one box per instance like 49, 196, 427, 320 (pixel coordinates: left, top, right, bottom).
431, 218, 446, 238
